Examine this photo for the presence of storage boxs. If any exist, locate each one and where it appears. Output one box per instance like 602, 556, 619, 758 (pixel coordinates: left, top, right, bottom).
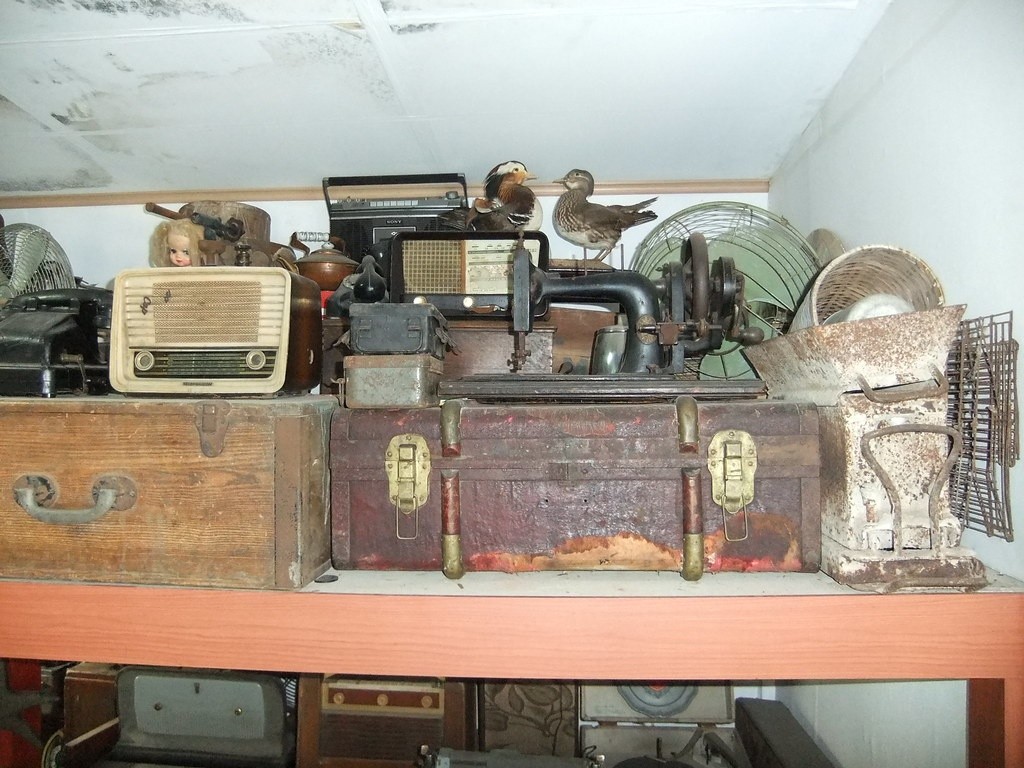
339, 355, 445, 409
324, 399, 824, 577
323, 317, 561, 390
346, 301, 451, 362
0, 390, 343, 589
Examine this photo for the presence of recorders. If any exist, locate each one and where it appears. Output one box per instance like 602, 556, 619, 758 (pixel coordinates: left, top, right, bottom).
322, 173, 470, 264
108, 266, 324, 399
392, 230, 550, 320
295, 671, 478, 768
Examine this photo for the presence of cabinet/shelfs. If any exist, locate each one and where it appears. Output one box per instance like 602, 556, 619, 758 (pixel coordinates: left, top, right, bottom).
0, 578, 1021, 766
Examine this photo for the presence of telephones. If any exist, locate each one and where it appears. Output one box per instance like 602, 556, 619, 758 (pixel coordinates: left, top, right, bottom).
0, 288, 114, 399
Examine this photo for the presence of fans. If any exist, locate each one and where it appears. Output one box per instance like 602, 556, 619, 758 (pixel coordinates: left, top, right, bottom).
629, 201, 828, 381
0, 224, 77, 321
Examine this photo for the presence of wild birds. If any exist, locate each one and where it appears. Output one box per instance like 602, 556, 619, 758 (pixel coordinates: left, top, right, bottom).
437, 161, 544, 232
552, 168, 658, 262
